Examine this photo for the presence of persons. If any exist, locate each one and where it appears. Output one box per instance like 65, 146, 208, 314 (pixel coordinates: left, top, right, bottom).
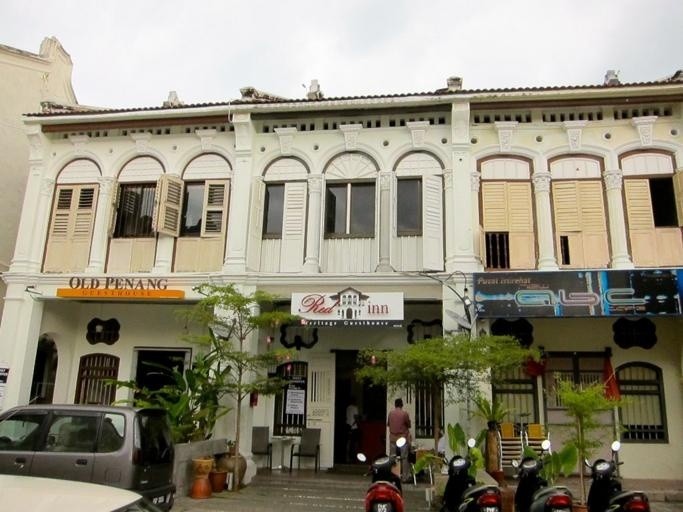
343, 396, 360, 462
386, 398, 411, 482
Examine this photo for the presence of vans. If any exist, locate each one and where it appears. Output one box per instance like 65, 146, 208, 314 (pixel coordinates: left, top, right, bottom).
0, 405, 175, 512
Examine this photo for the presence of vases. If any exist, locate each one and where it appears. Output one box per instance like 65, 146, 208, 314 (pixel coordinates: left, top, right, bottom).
189, 440, 247, 500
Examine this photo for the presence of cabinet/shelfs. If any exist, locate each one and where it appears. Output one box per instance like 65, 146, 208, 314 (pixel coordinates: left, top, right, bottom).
496, 429, 553, 479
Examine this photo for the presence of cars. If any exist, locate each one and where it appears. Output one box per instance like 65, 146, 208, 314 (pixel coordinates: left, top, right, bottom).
0, 474, 165, 512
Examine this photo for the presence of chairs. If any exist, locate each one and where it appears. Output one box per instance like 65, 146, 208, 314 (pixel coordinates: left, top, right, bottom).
413, 449, 434, 487
251, 426, 321, 475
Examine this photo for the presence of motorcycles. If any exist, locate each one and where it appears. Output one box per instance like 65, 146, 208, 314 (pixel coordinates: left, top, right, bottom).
440, 438, 501, 512
584, 440, 649, 512
512, 440, 572, 512
356, 436, 406, 512
